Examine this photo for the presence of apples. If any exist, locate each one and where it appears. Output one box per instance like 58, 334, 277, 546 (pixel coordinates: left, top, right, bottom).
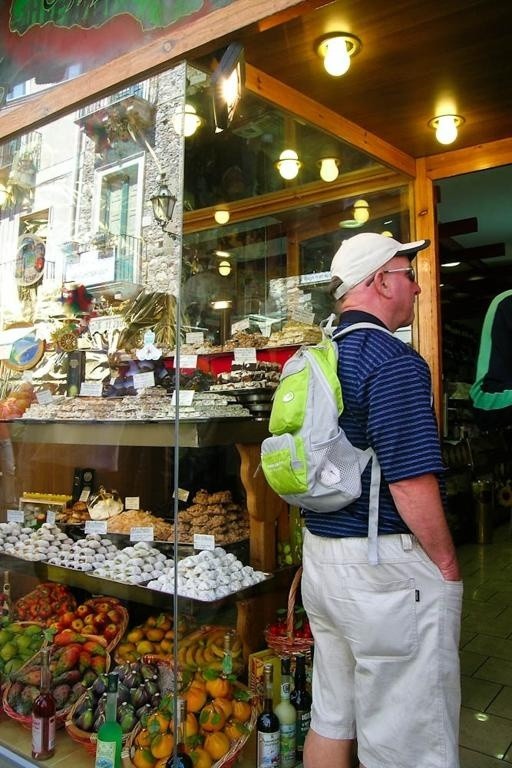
0, 616, 44, 676
60, 598, 121, 641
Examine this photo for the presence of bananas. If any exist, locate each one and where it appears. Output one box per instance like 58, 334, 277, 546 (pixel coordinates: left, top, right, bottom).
177, 627, 245, 674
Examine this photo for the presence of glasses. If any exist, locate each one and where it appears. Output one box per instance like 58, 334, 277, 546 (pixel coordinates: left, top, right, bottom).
366, 267, 414, 287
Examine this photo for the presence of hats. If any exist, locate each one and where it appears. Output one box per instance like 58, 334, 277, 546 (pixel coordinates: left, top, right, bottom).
331, 231, 431, 300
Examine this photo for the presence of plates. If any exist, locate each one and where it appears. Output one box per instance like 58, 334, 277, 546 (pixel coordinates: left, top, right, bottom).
1, 321, 44, 371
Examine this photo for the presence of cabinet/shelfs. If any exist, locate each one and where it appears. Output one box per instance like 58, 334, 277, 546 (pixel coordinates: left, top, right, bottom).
0, 382, 284, 766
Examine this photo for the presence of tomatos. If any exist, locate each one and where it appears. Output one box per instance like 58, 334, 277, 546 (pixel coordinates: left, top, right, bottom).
11, 584, 69, 624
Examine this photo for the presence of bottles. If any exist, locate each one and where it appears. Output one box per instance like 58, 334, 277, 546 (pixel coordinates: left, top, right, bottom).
3, 570, 12, 612
96, 673, 123, 768
166, 699, 192, 768
257, 664, 279, 767
273, 658, 297, 768
290, 652, 311, 760
223, 636, 232, 674
31, 651, 56, 760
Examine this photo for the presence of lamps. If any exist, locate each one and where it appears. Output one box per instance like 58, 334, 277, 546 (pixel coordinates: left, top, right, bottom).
171, 112, 206, 139
312, 28, 363, 77
427, 113, 466, 145
274, 160, 302, 180
207, 41, 246, 130
150, 173, 177, 233
315, 157, 343, 183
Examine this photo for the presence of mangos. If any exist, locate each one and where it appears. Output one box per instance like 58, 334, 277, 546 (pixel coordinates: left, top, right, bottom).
8, 632, 107, 716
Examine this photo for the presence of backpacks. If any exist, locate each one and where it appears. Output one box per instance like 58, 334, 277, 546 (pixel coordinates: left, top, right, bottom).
260, 312, 400, 565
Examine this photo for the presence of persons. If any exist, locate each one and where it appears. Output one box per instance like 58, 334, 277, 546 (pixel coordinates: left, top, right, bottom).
300, 231, 463, 768
470, 288, 512, 432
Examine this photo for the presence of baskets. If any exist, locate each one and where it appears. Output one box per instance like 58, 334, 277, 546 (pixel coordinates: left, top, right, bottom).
3, 639, 111, 728
13, 581, 75, 625
44, 596, 130, 651
114, 610, 198, 663
66, 653, 184, 756
121, 678, 258, 766
262, 564, 315, 659
0, 620, 49, 688
